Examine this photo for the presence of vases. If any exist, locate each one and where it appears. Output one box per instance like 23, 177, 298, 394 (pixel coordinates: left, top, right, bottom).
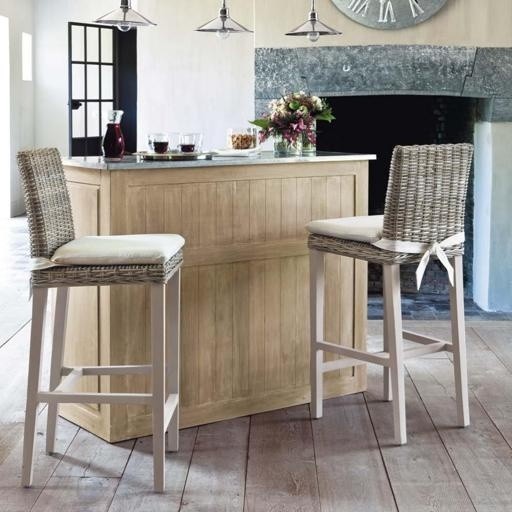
267, 121, 322, 155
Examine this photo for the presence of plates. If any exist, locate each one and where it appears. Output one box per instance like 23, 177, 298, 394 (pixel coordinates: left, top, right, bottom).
131, 151, 219, 161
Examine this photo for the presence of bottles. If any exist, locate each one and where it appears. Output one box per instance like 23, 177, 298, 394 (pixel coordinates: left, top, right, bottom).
100, 109, 124, 161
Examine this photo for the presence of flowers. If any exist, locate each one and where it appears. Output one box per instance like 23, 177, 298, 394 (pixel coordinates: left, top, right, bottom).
247, 88, 336, 146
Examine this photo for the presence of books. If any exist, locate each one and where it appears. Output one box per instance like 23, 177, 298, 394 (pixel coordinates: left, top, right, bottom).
142, 152, 198, 161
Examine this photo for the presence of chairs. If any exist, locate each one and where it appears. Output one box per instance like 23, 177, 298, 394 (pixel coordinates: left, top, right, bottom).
299, 143, 476, 448
14, 144, 184, 492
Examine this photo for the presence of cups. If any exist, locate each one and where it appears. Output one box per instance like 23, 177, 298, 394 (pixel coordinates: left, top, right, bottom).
148, 134, 169, 154
227, 127, 257, 148
179, 133, 202, 153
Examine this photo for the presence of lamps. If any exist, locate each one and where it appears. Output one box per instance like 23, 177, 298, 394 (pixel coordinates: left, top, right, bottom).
92, 0, 343, 42
